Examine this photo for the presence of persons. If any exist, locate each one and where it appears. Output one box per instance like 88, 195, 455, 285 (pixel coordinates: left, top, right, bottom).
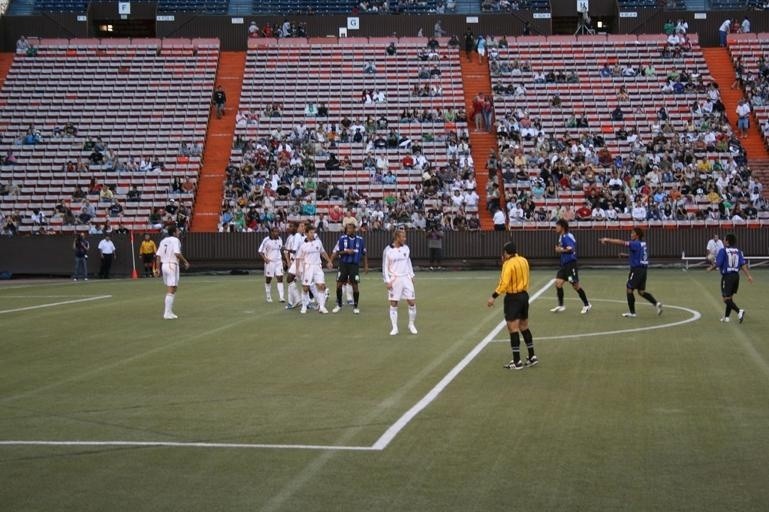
26, 43, 37, 57
217, 1, 481, 232
425, 227, 443, 270
1, 122, 202, 232
212, 84, 227, 118
97, 235, 116, 278
706, 236, 724, 271
138, 233, 157, 278
330, 222, 369, 316
16, 35, 29, 49
382, 230, 419, 335
707, 234, 753, 324
155, 225, 190, 319
550, 218, 593, 316
258, 227, 286, 303
72, 233, 90, 282
296, 226, 332, 315
284, 222, 320, 309
464, 1, 769, 232
487, 241, 538, 370
599, 228, 663, 317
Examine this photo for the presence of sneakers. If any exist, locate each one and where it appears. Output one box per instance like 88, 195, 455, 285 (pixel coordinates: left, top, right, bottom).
266, 298, 273, 303
318, 308, 329, 314
655, 301, 663, 314
549, 304, 567, 313
525, 354, 539, 368
407, 323, 418, 335
332, 305, 342, 313
293, 298, 301, 308
300, 306, 308, 314
279, 298, 286, 302
581, 303, 592, 315
719, 316, 730, 322
284, 304, 294, 309
172, 313, 178, 319
503, 358, 524, 369
352, 307, 359, 314
738, 309, 745, 322
389, 328, 399, 335
163, 315, 174, 320
622, 312, 636, 317
